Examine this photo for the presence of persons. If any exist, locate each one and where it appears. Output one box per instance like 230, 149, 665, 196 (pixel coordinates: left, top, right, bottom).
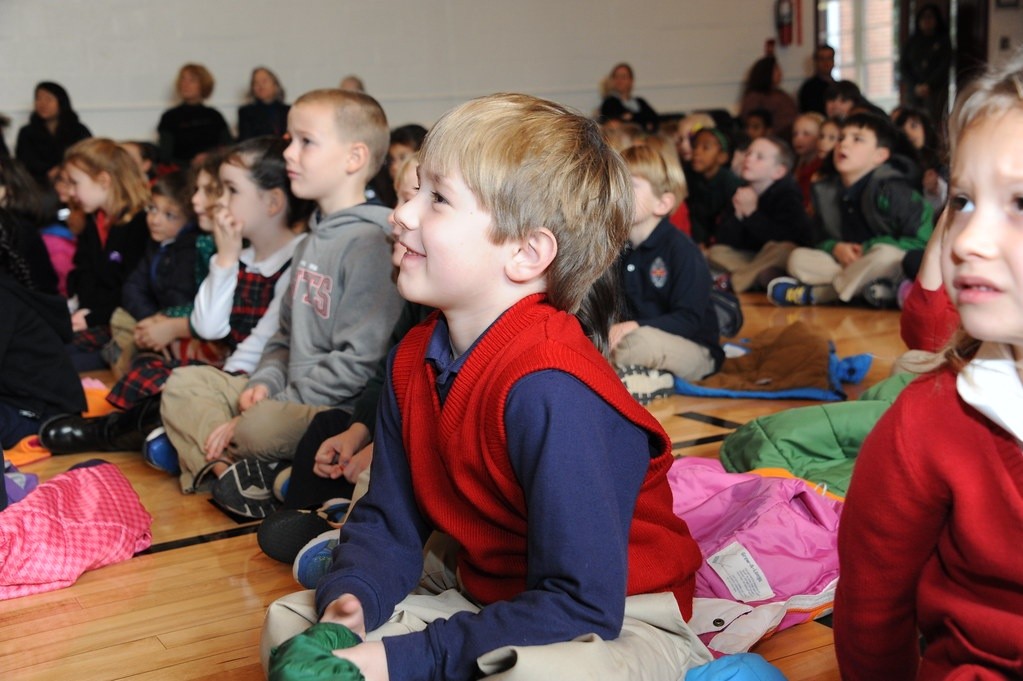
0, 81, 319, 478
341, 75, 365, 89
235, 68, 293, 143
161, 89, 433, 585
386, 123, 432, 188
597, 22, 956, 384
156, 65, 236, 171
260, 90, 717, 681
828, 52, 1023, 681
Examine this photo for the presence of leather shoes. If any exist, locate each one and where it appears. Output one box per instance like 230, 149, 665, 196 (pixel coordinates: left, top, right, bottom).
38, 413, 108, 455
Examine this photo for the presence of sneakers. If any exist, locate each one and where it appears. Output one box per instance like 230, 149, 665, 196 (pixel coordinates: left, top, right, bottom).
767, 277, 815, 306
143, 425, 180, 473
213, 458, 293, 518
865, 278, 899, 307
292, 528, 343, 586
256, 502, 351, 564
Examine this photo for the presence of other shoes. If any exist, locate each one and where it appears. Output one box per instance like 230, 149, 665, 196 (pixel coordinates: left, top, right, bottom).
618, 364, 681, 403
